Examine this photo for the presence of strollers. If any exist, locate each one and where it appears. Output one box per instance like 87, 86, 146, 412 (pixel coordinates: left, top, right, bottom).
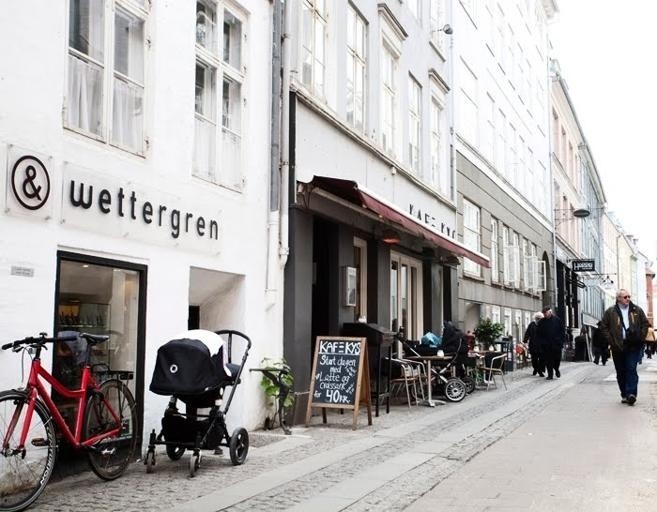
396, 332, 475, 402
144, 329, 251, 478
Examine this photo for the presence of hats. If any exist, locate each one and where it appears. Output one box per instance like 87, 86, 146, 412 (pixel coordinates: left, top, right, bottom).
542, 305, 551, 313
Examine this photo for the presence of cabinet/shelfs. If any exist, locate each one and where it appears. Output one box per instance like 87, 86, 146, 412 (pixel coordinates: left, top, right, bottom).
341, 322, 397, 417
54, 301, 111, 440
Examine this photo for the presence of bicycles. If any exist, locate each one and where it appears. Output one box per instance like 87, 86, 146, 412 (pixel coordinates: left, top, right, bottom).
0, 332, 139, 507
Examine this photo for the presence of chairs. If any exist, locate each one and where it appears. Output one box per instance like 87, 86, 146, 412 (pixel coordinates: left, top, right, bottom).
480, 352, 508, 391
399, 359, 427, 402
385, 357, 418, 408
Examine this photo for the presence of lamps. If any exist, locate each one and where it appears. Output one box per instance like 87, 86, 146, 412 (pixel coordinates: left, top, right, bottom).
382, 230, 399, 245
447, 256, 459, 266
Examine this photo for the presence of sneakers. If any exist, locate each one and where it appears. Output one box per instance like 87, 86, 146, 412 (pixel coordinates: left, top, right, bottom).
546, 377, 553, 380
533, 369, 537, 375
538, 372, 544, 377
556, 370, 560, 377
621, 397, 627, 403
627, 394, 636, 405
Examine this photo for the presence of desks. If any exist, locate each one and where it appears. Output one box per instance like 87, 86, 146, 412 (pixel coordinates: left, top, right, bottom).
403, 355, 453, 407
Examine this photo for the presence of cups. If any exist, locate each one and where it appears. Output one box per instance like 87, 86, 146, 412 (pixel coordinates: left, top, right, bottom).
436, 350, 445, 357
473, 345, 480, 352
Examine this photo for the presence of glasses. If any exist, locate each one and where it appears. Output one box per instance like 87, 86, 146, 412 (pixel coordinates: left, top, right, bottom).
619, 296, 630, 299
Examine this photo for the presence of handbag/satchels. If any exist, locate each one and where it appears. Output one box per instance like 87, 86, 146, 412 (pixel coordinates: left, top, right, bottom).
626, 326, 645, 350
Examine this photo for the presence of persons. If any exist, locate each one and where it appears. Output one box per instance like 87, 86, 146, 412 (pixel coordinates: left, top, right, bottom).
523, 312, 545, 377
592, 289, 656, 406
536, 307, 565, 380
